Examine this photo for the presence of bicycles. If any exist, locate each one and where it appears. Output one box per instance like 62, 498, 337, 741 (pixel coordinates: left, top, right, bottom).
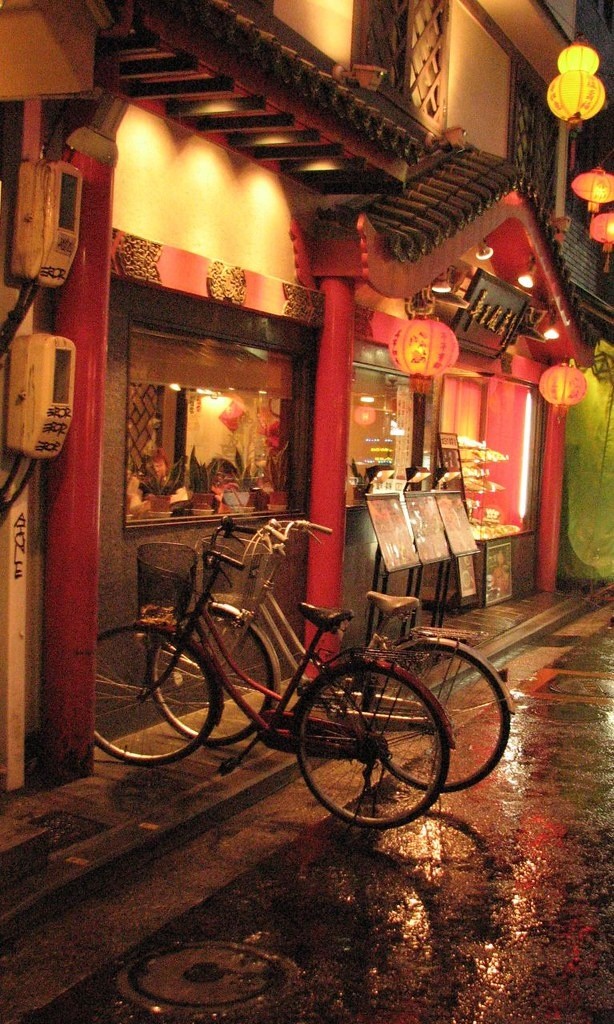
141, 517, 516, 795
92, 517, 458, 828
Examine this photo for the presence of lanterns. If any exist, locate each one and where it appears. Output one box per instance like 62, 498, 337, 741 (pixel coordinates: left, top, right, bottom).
547, 43, 605, 129
539, 362, 588, 414
388, 314, 460, 393
353, 404, 376, 426
589, 212, 614, 252
571, 168, 614, 211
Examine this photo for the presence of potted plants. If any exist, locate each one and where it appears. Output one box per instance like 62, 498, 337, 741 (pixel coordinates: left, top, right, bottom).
135, 454, 186, 512
258, 440, 294, 506
217, 448, 262, 505
348, 457, 374, 501
184, 445, 225, 510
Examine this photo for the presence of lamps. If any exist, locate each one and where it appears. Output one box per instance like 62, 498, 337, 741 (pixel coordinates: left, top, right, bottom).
538, 304, 558, 341
425, 123, 468, 154
432, 267, 453, 295
404, 466, 431, 492
331, 63, 389, 92
361, 464, 396, 500
475, 239, 494, 261
433, 467, 461, 491
516, 252, 538, 289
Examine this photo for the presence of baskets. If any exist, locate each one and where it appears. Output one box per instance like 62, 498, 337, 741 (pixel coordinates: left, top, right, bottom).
202, 535, 272, 613
137, 542, 198, 635
